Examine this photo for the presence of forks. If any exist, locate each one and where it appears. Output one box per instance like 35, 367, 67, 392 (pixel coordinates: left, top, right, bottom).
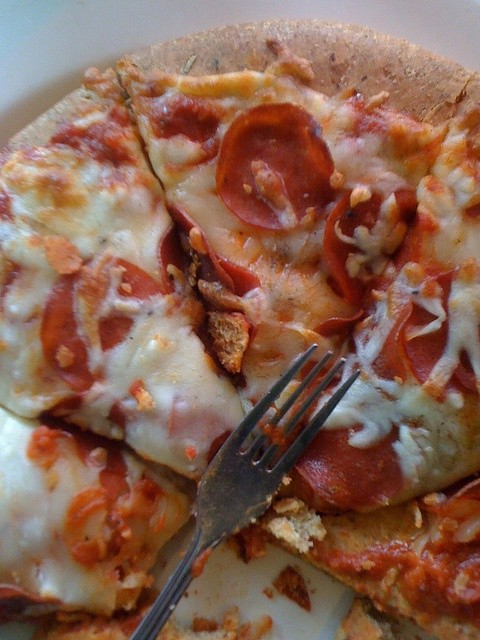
125, 340, 361, 640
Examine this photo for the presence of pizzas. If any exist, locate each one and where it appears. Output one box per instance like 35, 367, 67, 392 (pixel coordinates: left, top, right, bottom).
1, 18, 479, 639
